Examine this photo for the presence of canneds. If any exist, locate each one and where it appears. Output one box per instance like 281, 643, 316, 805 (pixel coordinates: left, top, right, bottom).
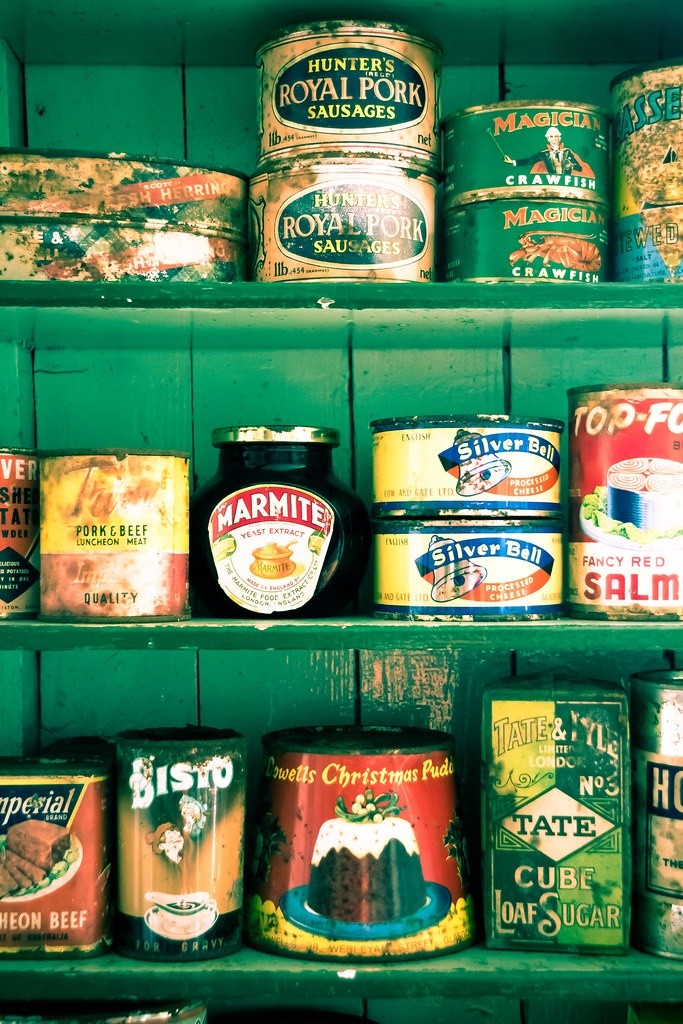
0, 22, 682, 964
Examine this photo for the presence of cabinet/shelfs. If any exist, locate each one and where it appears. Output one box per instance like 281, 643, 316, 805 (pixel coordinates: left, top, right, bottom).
1, 1, 683, 1024
